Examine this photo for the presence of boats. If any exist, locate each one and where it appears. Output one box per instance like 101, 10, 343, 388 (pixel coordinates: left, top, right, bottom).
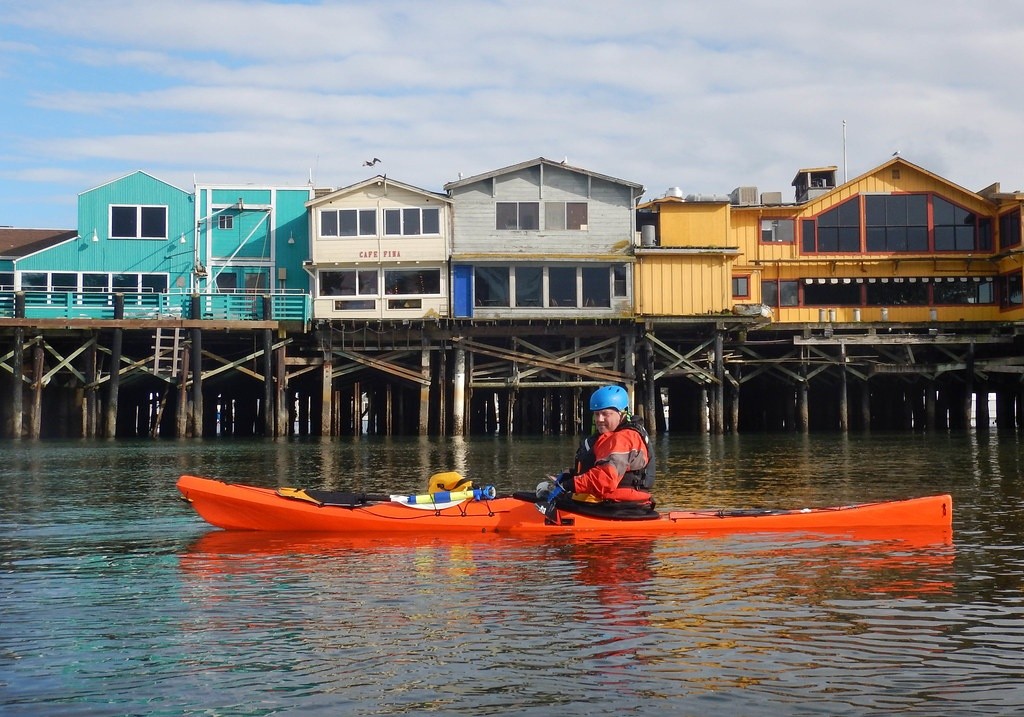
175, 474, 954, 533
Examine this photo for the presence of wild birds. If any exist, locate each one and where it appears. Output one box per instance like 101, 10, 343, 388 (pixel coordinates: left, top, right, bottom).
362, 157, 382, 167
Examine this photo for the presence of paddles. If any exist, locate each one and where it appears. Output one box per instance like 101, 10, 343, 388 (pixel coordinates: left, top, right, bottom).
274, 484, 498, 509
534, 466, 570, 526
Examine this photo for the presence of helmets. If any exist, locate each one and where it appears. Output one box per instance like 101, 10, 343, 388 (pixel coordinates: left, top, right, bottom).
590, 386, 629, 414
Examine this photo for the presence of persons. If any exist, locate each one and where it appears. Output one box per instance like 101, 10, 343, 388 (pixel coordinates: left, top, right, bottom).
548, 386, 656, 511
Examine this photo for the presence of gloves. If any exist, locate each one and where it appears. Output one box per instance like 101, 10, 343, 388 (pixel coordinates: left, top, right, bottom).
547, 486, 562, 503
548, 471, 565, 484
